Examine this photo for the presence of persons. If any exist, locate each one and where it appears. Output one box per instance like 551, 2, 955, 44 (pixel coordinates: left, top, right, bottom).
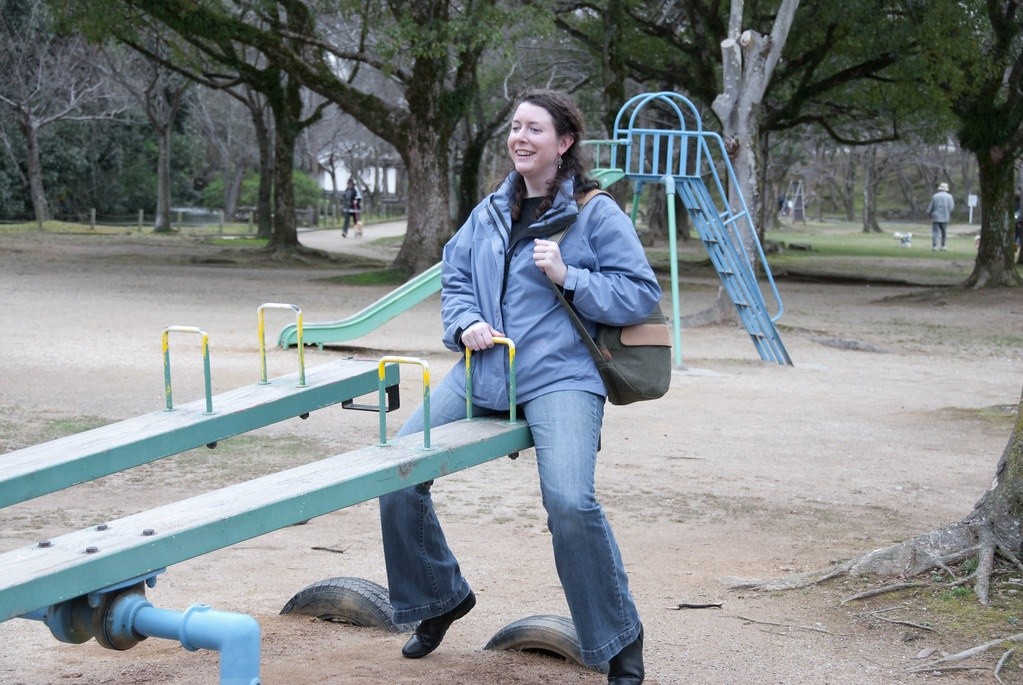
340, 178, 363, 238
1014, 185, 1023, 252
927, 183, 954, 251
776, 191, 793, 215
377, 88, 660, 685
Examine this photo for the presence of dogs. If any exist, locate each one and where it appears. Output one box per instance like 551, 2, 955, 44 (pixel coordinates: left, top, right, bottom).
893, 231, 912, 248
354, 221, 364, 238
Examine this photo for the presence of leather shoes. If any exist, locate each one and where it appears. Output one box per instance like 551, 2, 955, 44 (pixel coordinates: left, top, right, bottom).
607, 621, 644, 685
402, 589, 476, 659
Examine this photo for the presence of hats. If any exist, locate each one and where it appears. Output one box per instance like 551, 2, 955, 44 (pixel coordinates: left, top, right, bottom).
937, 183, 949, 191
347, 179, 355, 185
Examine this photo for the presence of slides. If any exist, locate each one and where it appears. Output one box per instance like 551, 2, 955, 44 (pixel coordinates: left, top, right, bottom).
276, 166, 626, 348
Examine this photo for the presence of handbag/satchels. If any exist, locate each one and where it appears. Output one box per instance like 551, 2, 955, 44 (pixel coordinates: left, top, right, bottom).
543, 189, 672, 405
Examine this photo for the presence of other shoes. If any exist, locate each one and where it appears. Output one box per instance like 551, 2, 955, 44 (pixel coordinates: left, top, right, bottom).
940, 247, 948, 251
932, 246, 937, 251
342, 234, 346, 237
